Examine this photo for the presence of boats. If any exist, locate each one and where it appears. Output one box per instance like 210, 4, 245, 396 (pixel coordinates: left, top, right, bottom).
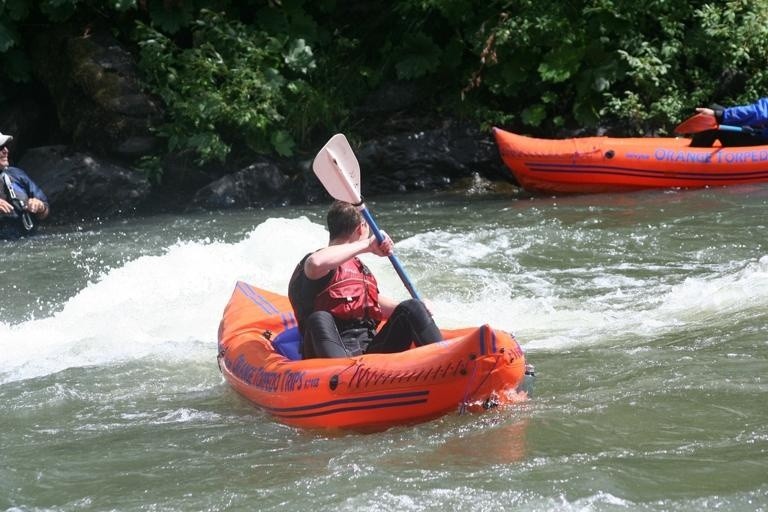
490, 123, 767, 195
216, 281, 537, 434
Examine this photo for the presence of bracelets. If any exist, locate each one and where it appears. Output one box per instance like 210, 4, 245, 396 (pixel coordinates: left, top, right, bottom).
714, 109, 723, 122
38, 207, 46, 214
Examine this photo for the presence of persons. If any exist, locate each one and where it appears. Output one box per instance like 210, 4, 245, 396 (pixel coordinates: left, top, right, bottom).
688, 96, 768, 149
286, 199, 443, 359
0, 131, 51, 241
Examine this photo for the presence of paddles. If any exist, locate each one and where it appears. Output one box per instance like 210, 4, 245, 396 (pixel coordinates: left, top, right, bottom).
675, 113, 764, 133
312, 133, 435, 323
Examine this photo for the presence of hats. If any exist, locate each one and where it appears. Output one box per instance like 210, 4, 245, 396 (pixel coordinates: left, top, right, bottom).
0, 132, 13, 147
